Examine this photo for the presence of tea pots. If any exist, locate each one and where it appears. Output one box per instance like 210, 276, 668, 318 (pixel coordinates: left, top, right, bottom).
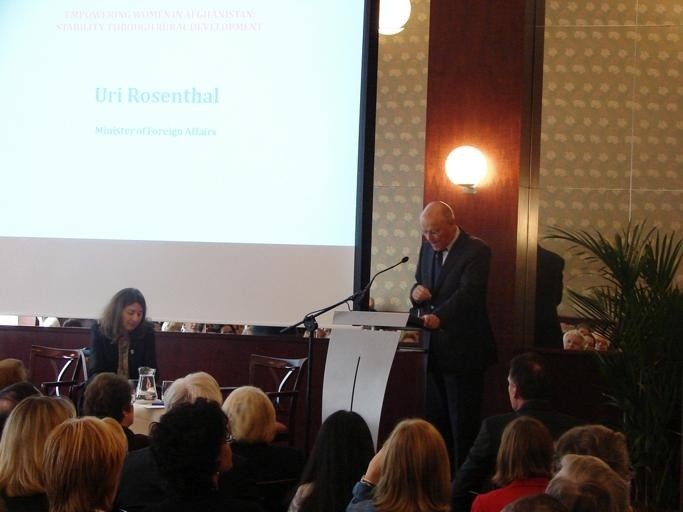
135, 366, 157, 401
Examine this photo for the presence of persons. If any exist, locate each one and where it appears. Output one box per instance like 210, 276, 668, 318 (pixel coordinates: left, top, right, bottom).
563, 324, 611, 352
147, 396, 269, 512
408, 198, 501, 485
285, 409, 379, 511
83, 285, 161, 405
42, 415, 129, 512
82, 373, 151, 453
471, 423, 636, 512
451, 347, 568, 492
221, 385, 305, 480
469, 415, 556, 512
1, 359, 44, 429
536, 240, 568, 352
347, 418, 456, 512
1, 397, 77, 512
162, 369, 222, 421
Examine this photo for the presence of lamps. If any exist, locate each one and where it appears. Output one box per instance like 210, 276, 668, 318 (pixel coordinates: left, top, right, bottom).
446, 143, 487, 197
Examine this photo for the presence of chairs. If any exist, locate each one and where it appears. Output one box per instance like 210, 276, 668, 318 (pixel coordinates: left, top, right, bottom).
220, 353, 307, 424
23, 343, 83, 400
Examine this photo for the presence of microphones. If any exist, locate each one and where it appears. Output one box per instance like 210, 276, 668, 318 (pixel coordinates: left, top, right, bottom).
353, 257, 409, 310
350, 289, 362, 301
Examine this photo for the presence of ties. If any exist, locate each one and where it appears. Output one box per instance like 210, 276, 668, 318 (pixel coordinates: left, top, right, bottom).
433, 252, 442, 291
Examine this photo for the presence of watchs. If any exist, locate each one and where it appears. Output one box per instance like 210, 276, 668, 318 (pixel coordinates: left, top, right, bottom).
357, 473, 378, 491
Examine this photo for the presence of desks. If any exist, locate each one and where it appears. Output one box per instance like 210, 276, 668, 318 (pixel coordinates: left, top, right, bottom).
132, 393, 164, 437
